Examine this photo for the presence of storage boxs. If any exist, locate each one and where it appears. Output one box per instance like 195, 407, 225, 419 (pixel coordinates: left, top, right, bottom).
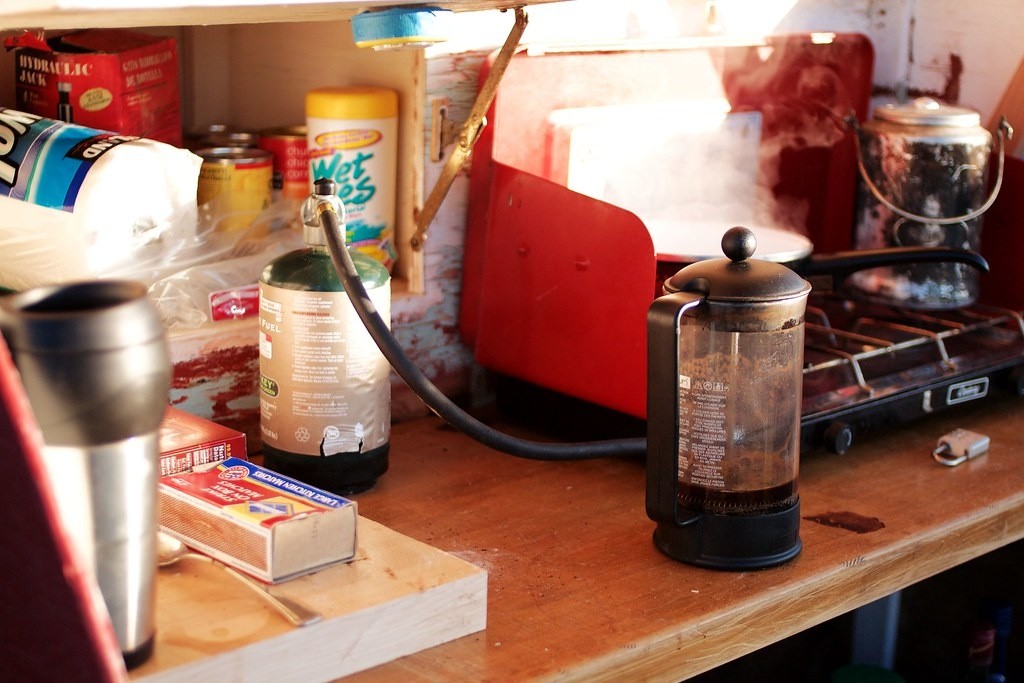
3, 28, 182, 150
159, 406, 358, 584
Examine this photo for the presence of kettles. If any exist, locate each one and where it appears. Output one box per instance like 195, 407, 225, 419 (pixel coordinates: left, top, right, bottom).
845, 96, 1013, 308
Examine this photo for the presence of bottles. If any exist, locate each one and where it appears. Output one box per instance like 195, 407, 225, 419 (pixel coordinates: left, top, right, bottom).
258, 178, 392, 499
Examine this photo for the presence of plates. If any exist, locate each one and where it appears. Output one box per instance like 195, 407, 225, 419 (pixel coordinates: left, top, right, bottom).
654, 225, 990, 287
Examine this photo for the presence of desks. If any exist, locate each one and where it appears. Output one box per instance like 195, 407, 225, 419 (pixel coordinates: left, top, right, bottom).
252, 390, 1023, 683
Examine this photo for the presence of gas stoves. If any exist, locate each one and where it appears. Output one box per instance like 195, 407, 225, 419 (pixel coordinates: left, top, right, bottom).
460, 33, 1024, 468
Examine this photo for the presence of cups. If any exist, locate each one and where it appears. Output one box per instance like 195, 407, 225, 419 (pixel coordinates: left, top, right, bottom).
643, 225, 812, 570
0, 280, 170, 440
40, 431, 161, 670
